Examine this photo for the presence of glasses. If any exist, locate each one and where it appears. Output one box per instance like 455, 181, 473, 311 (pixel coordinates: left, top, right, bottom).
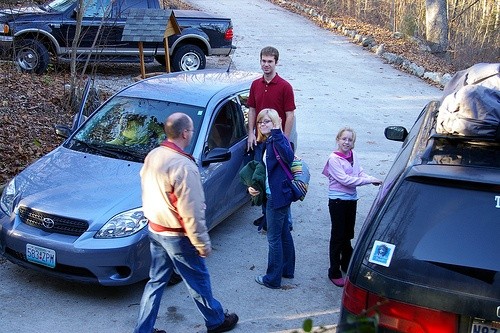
258, 120, 272, 126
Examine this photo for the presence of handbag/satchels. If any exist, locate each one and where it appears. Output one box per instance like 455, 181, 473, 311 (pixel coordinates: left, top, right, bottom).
291, 157, 310, 200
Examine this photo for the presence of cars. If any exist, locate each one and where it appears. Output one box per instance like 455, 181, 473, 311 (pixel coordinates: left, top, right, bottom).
0, 69, 298, 287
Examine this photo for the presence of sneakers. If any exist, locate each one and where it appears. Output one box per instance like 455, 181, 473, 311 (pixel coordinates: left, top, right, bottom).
153, 328, 166, 333
208, 309, 237, 333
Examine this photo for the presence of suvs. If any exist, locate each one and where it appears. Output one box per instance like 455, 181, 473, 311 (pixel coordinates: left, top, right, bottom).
335, 99, 500, 333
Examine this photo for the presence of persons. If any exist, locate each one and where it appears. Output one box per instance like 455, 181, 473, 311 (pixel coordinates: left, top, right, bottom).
246, 46, 296, 231
134, 112, 239, 333
248, 108, 296, 290
321, 126, 382, 287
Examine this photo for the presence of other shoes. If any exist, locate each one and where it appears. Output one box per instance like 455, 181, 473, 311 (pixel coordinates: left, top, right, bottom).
255, 275, 264, 284
331, 276, 345, 287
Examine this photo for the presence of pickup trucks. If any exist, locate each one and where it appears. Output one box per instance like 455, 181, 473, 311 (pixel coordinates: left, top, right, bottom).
0, 0, 237, 76
